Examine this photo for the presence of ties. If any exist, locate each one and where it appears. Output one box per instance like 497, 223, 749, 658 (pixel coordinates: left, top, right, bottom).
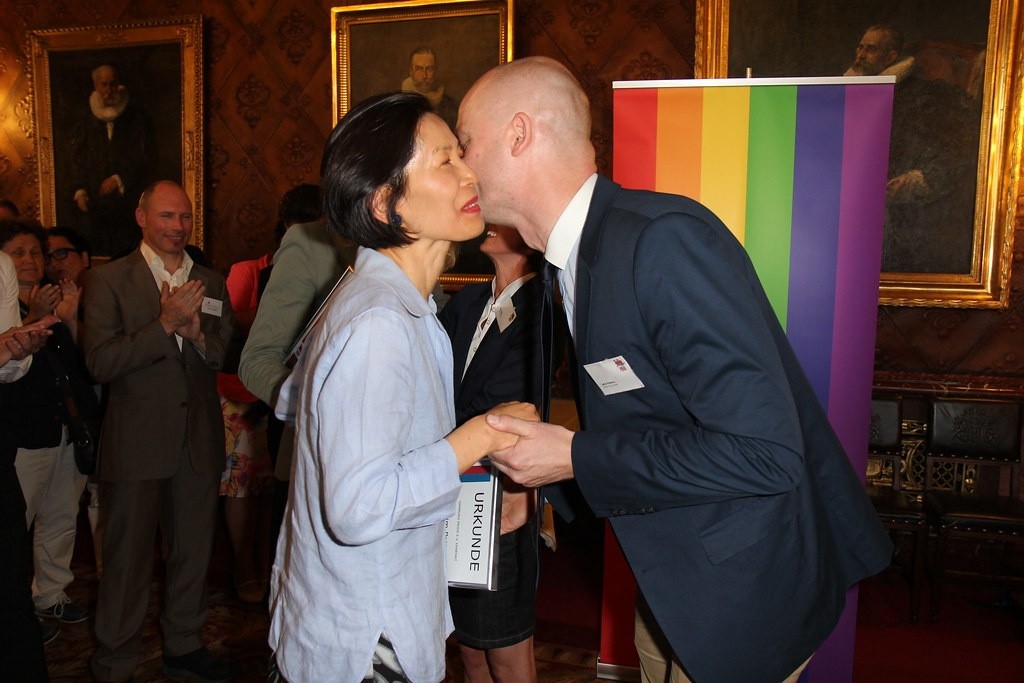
516, 262, 554, 596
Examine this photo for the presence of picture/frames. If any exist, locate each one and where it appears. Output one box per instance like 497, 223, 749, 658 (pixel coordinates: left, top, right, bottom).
692, 0, 1024, 312
331, 0, 514, 290
24, 13, 204, 268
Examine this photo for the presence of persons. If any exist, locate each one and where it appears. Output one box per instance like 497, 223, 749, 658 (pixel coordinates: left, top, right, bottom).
0, 180, 328, 683
437, 224, 550, 682
237, 213, 457, 558
268, 90, 541, 683
454, 56, 898, 683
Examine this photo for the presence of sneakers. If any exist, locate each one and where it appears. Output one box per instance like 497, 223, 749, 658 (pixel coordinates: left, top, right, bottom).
39, 623, 61, 645
34, 595, 90, 623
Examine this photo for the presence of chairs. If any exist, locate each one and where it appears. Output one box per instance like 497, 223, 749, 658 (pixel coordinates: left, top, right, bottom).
865, 394, 928, 624
921, 395, 1023, 622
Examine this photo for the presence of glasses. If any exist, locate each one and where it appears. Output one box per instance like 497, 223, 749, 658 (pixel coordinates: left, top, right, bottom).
44, 248, 77, 265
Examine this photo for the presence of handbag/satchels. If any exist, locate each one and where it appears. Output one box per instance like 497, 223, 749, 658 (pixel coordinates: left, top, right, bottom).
68, 418, 100, 475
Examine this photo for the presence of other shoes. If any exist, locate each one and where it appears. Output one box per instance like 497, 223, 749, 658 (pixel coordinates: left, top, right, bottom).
127, 675, 133, 683
162, 647, 236, 683
230, 573, 268, 620
